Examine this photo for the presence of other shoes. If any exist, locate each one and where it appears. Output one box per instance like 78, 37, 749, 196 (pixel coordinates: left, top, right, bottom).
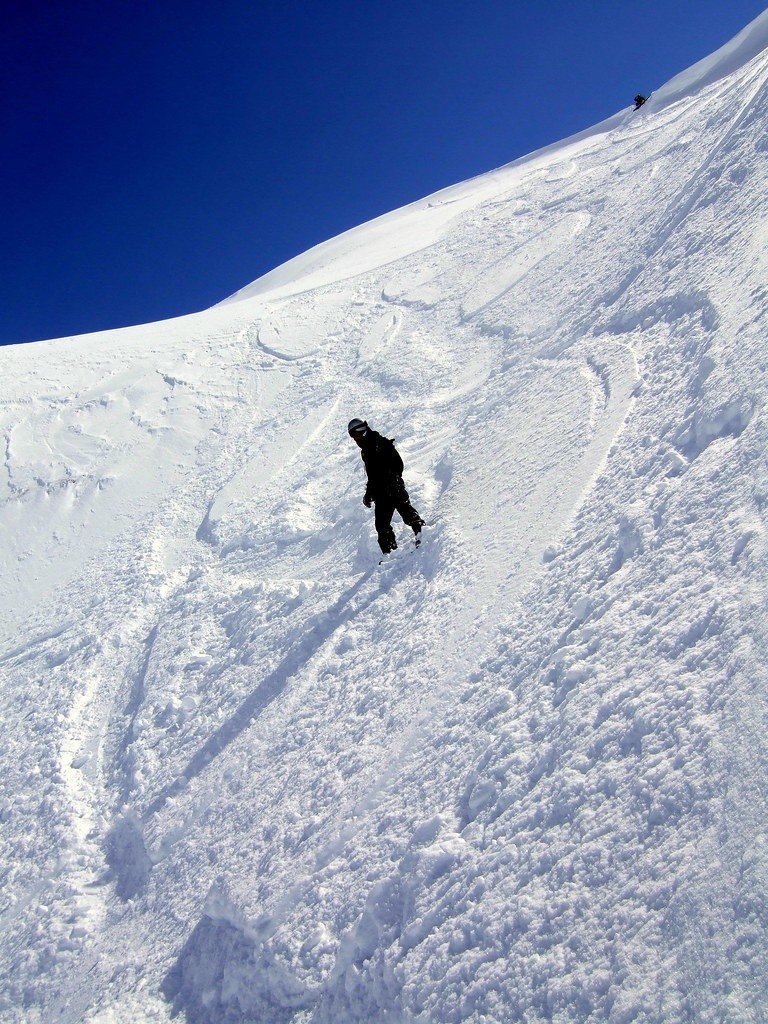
379, 561, 383, 564
415, 540, 421, 549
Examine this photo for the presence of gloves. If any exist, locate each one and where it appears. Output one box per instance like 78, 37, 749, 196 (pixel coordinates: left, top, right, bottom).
363, 492, 372, 508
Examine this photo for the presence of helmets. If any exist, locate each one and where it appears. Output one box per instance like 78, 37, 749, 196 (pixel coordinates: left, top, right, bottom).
348, 419, 369, 437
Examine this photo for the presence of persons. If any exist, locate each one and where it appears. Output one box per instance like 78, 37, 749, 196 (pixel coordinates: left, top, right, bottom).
634, 94, 645, 107
348, 418, 426, 565
